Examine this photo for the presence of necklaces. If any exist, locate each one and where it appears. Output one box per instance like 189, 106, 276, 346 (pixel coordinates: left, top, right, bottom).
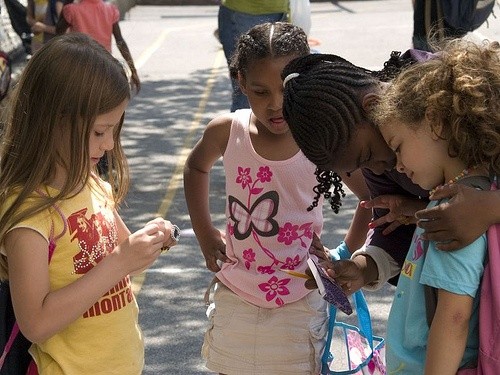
429, 165, 481, 195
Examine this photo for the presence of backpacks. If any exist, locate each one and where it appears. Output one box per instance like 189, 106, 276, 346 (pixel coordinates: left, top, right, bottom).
425, 175, 500, 375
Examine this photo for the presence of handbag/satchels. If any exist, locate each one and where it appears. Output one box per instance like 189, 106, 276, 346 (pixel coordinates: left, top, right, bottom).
0, 229, 55, 375
321, 240, 385, 375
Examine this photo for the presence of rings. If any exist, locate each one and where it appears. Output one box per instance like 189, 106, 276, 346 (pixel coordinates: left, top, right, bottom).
170, 224, 180, 242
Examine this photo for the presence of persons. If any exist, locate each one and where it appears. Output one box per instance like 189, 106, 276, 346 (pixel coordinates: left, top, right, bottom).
27, 0, 141, 95
183, 21, 372, 375
369, 20, 500, 375
218, 0, 311, 112
0, 34, 181, 375
280, 49, 500, 296
